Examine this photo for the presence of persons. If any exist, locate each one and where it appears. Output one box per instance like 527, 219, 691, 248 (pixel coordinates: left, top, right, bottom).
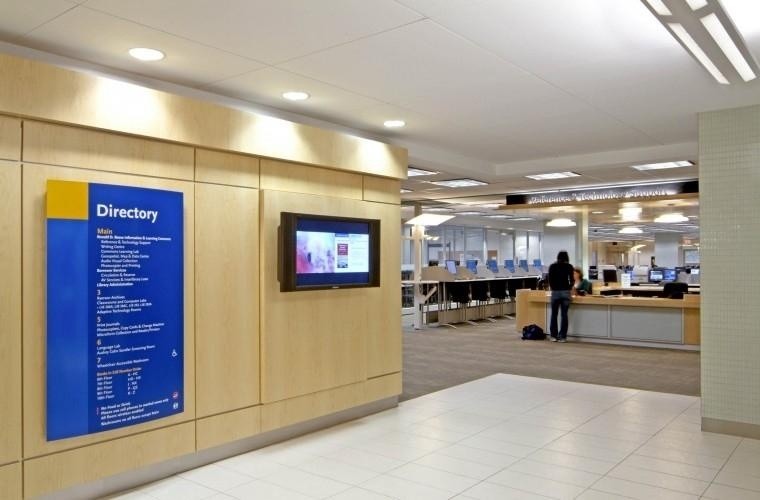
573, 268, 592, 295
548, 252, 575, 343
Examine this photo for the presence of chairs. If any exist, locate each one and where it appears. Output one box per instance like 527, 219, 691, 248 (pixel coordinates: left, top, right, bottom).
540, 273, 549, 290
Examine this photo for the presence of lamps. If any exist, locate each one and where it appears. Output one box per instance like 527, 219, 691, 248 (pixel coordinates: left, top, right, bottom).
643, 0, 760, 88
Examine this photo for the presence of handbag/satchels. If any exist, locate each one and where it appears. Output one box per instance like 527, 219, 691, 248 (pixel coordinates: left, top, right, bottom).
521, 324, 547, 340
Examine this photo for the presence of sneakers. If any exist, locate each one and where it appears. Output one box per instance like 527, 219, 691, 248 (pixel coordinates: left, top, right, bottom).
550, 336, 569, 343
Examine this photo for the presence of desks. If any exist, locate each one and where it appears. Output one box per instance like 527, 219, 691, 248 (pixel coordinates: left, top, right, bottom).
401, 264, 540, 330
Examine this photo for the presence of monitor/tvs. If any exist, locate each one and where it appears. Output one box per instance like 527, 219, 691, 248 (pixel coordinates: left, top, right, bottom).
589, 262, 701, 286
426, 257, 544, 275
277, 210, 381, 292
